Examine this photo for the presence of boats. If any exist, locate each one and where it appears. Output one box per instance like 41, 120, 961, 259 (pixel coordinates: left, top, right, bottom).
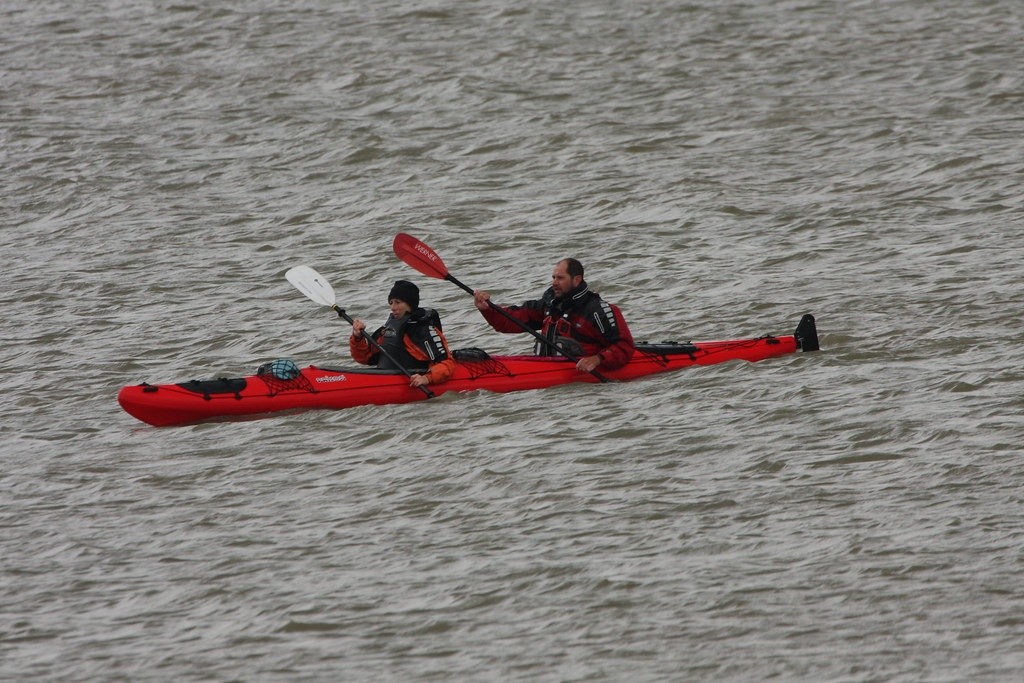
118, 312, 821, 428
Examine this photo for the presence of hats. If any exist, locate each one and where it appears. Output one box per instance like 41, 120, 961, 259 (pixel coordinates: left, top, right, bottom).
388, 280, 419, 311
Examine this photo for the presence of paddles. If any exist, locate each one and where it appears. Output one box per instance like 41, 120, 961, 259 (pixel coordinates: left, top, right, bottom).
284, 260, 439, 400
392, 230, 610, 384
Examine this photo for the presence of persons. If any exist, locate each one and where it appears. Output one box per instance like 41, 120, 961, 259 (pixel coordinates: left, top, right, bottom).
473, 257, 635, 372
349, 281, 455, 388
